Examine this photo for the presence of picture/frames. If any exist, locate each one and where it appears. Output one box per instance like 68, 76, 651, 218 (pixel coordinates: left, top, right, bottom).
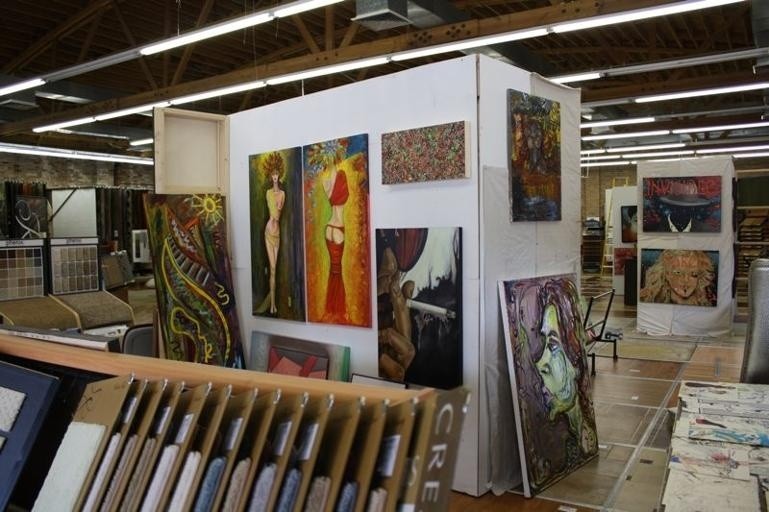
248, 332, 349, 386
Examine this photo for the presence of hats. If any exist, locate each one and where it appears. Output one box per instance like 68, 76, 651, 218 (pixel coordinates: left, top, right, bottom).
659, 180, 710, 206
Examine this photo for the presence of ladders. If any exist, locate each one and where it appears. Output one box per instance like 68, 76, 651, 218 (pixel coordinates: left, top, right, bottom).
600, 176, 629, 280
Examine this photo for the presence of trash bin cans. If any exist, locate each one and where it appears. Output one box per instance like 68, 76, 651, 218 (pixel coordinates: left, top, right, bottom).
624, 256, 637, 306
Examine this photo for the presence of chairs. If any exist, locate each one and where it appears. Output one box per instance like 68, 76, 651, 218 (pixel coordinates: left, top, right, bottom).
119, 325, 155, 356
